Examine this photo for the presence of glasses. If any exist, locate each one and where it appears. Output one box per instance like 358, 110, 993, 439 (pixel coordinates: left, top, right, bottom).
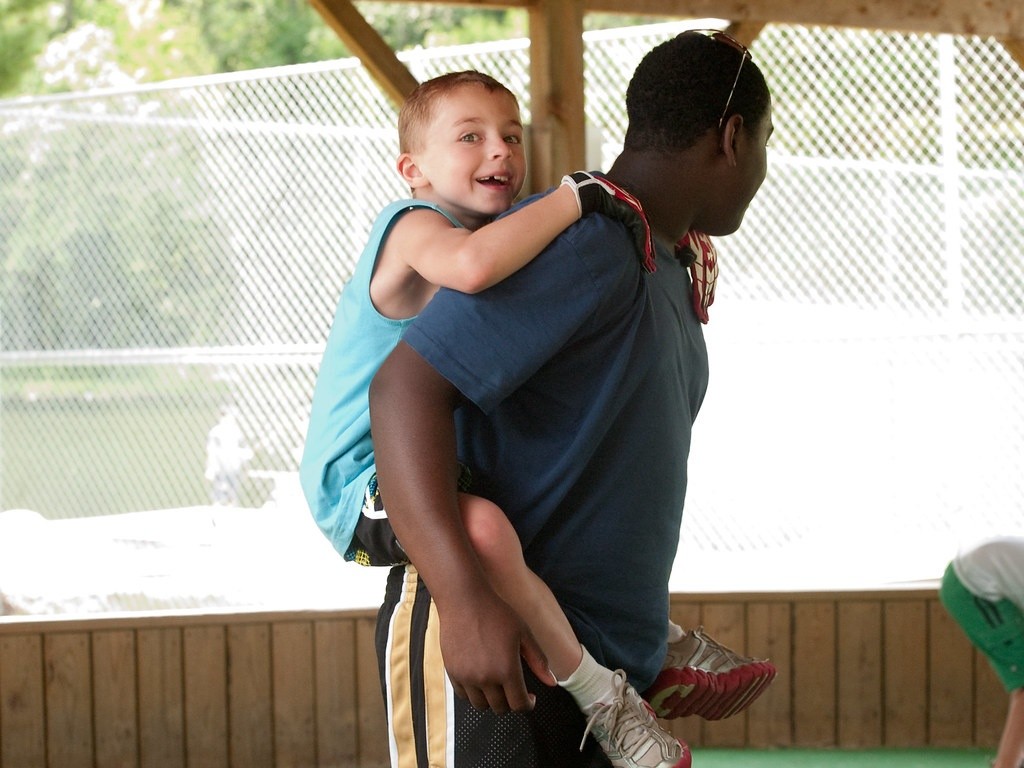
676, 28, 753, 129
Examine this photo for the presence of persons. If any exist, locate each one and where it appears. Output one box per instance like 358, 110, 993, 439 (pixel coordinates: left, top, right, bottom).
205, 408, 253, 507
367, 25, 772, 768
939, 535, 1023, 767
301, 70, 779, 768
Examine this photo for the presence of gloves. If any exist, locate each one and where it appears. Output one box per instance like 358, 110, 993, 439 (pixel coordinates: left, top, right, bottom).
560, 169, 657, 275
676, 227, 719, 325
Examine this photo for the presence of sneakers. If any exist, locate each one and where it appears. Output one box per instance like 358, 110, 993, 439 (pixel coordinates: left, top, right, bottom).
639, 624, 778, 722
579, 668, 692, 768
